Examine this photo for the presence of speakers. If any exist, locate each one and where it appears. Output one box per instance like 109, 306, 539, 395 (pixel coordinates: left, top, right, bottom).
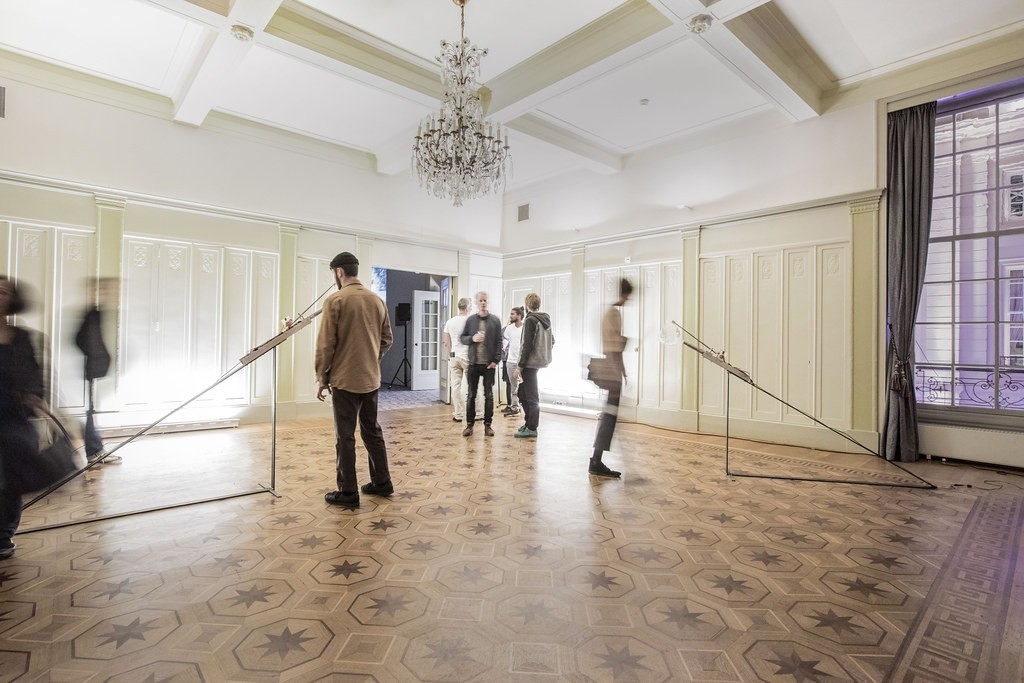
398, 303, 410, 322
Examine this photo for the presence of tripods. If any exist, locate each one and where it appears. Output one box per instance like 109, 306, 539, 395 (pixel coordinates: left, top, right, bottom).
388, 321, 411, 390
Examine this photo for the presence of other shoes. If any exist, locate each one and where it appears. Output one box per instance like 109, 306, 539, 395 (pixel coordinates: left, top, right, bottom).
453, 417, 462, 422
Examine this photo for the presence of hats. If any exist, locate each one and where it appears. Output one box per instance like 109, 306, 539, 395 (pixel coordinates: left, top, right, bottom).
330, 252, 359, 268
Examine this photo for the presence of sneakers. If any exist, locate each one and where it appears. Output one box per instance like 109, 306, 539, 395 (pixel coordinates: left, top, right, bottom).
588, 457, 621, 477
463, 425, 473, 436
514, 425, 537, 437
501, 407, 521, 417
485, 425, 494, 436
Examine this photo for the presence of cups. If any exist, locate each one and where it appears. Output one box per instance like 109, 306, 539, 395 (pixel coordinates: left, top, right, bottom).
477, 330, 485, 335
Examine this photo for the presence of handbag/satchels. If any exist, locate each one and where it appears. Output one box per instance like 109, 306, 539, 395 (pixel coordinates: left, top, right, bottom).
14, 435, 80, 494
588, 358, 622, 390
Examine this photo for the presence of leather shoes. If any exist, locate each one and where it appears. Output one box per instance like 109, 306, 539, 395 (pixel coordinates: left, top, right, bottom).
361, 483, 393, 496
325, 491, 359, 508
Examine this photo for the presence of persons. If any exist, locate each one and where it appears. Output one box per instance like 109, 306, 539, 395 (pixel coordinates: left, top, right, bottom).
445, 297, 485, 422
512, 293, 555, 438
459, 290, 503, 436
588, 278, 635, 477
1, 274, 47, 559
315, 252, 394, 509
500, 305, 526, 417
